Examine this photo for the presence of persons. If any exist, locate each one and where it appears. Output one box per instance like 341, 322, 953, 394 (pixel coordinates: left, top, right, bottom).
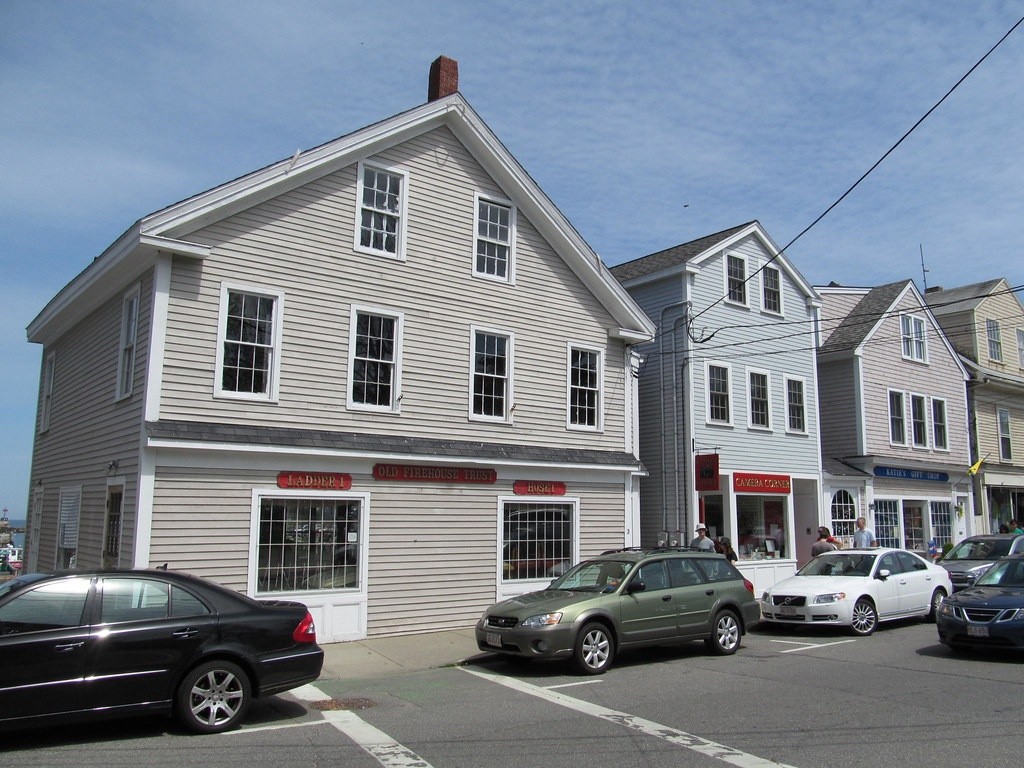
689, 523, 716, 552
717, 537, 738, 567
853, 518, 877, 547
810, 527, 843, 575
999, 519, 1024, 534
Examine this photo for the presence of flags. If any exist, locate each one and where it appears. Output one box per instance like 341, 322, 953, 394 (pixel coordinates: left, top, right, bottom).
967, 458, 986, 476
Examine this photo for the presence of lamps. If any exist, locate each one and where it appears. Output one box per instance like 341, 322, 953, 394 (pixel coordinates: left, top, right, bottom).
108, 460, 119, 470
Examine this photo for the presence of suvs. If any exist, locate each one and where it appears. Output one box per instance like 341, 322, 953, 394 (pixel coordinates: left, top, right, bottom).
475, 545, 763, 676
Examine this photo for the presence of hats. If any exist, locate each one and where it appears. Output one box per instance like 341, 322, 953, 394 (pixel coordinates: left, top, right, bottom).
717, 537, 730, 545
695, 523, 708, 532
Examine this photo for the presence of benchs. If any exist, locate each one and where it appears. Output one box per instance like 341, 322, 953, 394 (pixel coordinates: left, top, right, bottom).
885, 556, 915, 574
663, 571, 698, 587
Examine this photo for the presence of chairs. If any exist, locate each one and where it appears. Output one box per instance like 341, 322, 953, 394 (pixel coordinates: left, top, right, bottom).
866, 556, 885, 577
645, 569, 665, 590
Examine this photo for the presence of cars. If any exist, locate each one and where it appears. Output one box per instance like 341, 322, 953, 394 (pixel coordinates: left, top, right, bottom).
760, 547, 954, 636
1, 563, 325, 736
935, 552, 1024, 663
938, 533, 1024, 592
